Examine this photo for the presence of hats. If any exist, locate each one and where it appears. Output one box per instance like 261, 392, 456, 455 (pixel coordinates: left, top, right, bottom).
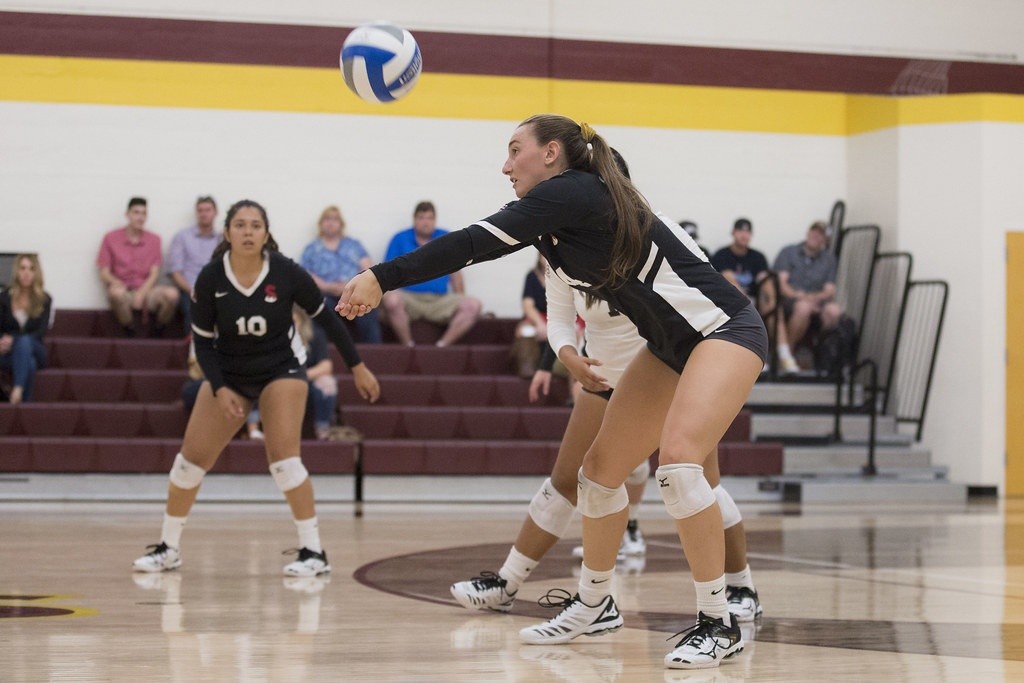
679, 223, 699, 238
734, 220, 752, 231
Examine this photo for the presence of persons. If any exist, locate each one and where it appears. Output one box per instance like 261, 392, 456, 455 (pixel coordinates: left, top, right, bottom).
167, 196, 381, 441
0, 254, 54, 406
384, 201, 482, 347
132, 200, 380, 578
516, 218, 843, 380
96, 199, 180, 338
335, 114, 769, 668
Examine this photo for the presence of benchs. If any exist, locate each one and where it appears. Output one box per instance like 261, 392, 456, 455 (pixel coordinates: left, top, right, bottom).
0, 306, 785, 476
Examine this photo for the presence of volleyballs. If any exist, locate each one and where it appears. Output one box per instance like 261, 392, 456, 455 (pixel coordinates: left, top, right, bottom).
339, 24, 425, 105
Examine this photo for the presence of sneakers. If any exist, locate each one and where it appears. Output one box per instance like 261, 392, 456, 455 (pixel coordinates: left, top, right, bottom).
450, 571, 519, 613
283, 547, 330, 577
132, 542, 182, 573
518, 588, 624, 645
725, 585, 763, 622
664, 611, 745, 670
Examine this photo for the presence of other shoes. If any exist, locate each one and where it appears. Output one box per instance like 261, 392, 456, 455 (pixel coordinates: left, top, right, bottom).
571, 529, 647, 561
777, 358, 801, 376
